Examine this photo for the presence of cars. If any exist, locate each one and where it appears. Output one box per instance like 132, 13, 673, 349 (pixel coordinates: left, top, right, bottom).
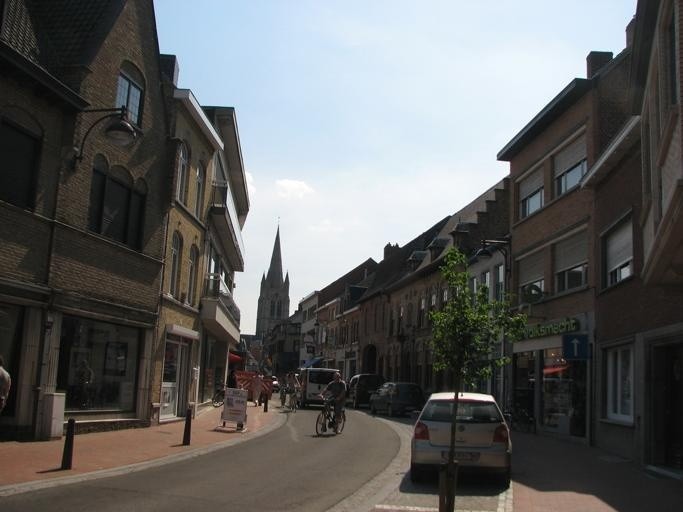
262, 376, 279, 393
407, 390, 512, 490
367, 382, 425, 419
516, 377, 573, 417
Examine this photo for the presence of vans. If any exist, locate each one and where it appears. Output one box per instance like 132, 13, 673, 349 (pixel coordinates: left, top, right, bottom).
294, 367, 340, 409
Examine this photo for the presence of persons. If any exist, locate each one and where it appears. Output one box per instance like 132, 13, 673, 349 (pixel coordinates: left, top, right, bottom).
0, 355, 11, 416
75, 358, 94, 408
278, 373, 287, 407
250, 370, 262, 407
287, 371, 301, 410
317, 371, 346, 434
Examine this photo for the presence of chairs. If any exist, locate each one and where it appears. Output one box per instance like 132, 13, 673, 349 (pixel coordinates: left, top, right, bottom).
431, 404, 450, 419
470, 406, 492, 421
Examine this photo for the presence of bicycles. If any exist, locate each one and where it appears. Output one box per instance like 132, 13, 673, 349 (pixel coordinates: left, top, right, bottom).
278, 385, 288, 408
496, 398, 536, 434
285, 386, 301, 414
76, 381, 96, 410
211, 382, 227, 407
314, 395, 345, 437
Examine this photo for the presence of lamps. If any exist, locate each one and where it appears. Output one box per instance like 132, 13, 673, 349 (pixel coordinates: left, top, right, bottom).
71, 105, 137, 167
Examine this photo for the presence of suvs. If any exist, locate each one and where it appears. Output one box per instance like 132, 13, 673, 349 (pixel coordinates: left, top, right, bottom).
344, 374, 384, 411
